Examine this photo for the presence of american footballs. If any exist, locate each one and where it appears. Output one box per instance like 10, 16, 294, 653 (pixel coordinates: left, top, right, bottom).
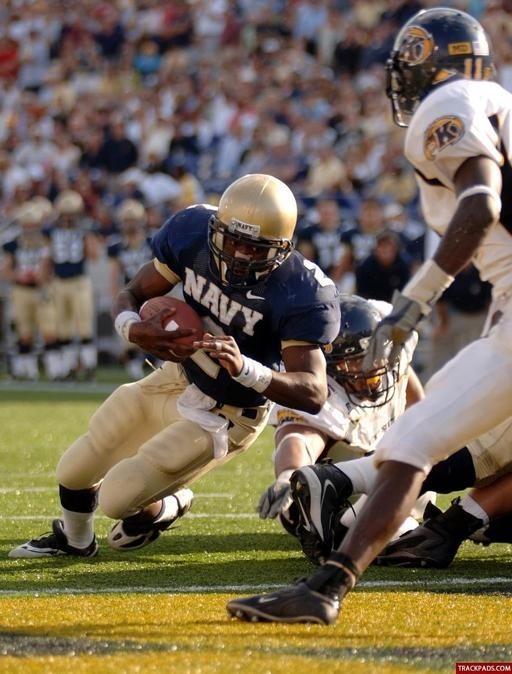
138, 298, 205, 358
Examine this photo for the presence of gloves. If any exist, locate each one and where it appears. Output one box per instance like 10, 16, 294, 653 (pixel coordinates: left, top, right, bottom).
256, 469, 294, 519
361, 289, 424, 374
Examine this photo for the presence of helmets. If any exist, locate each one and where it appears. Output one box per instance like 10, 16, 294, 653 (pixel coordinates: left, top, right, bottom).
207, 174, 298, 288
391, 8, 492, 102
320, 300, 383, 384
117, 200, 146, 223
14, 191, 83, 224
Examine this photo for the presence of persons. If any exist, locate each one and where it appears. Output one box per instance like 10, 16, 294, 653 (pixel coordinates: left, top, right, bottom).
0, 1, 511, 625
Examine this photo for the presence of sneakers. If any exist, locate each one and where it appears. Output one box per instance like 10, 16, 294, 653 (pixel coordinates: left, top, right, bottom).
123, 355, 145, 379
12, 343, 98, 381
280, 457, 512, 569
226, 576, 341, 625
8, 519, 99, 558
108, 487, 194, 550
